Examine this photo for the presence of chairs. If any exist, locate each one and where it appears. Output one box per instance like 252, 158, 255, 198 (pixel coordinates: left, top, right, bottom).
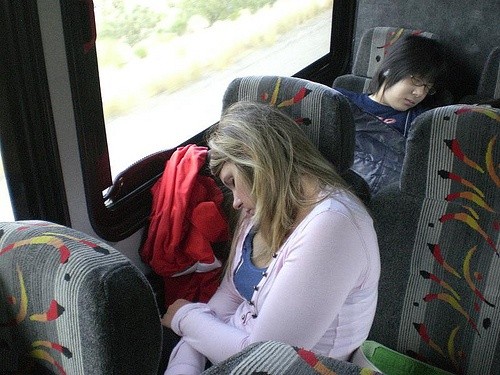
221, 76, 370, 204
332, 27, 453, 104
0, 220, 163, 375
368, 104, 500, 375
477, 46, 500, 100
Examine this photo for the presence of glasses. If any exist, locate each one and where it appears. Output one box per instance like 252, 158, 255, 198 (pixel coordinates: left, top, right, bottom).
408, 73, 437, 95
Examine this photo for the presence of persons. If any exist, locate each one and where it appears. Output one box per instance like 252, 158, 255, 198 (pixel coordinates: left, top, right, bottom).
155, 99, 381, 375
334, 33, 455, 139
